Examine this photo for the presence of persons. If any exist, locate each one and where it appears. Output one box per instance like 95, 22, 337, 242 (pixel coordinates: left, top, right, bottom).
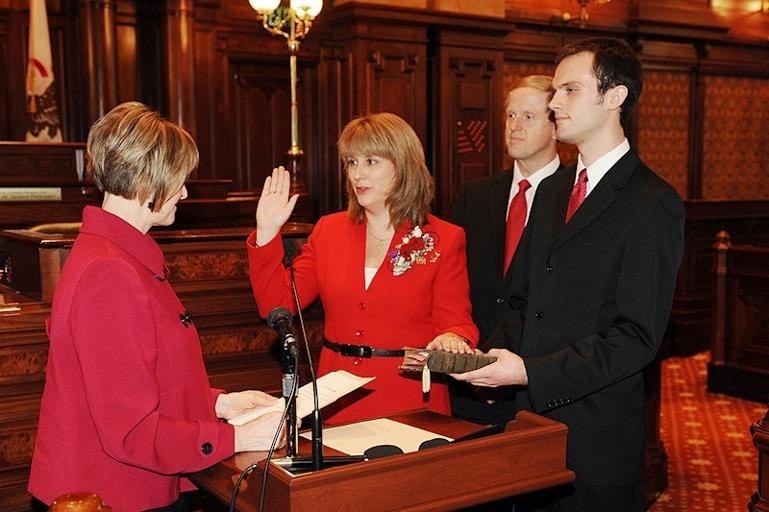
444, 33, 686, 511
243, 112, 481, 425
27, 100, 304, 512
451, 74, 568, 347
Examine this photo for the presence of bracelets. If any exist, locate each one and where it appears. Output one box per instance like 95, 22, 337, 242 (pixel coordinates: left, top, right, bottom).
256, 243, 260, 248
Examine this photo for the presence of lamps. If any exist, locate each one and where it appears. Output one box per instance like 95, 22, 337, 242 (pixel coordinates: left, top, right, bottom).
250, 0, 324, 51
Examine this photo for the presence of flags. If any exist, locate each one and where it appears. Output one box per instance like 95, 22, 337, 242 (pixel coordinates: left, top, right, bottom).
23, 0, 62, 144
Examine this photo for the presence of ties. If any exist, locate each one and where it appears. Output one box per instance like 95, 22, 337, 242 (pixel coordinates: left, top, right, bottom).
565, 169, 589, 225
501, 180, 532, 280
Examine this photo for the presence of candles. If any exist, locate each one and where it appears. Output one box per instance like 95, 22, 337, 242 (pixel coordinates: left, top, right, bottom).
288, 55, 299, 150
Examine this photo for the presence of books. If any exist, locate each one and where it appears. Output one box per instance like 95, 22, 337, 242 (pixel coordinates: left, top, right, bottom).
399, 343, 496, 374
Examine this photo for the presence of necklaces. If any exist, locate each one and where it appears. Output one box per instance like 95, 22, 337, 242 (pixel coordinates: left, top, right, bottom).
368, 227, 393, 247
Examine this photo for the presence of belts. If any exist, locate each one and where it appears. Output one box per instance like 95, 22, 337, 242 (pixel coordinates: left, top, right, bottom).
322, 338, 405, 357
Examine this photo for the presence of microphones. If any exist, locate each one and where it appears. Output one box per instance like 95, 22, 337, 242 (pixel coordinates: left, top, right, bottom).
267, 306, 299, 360
284, 237, 323, 471
